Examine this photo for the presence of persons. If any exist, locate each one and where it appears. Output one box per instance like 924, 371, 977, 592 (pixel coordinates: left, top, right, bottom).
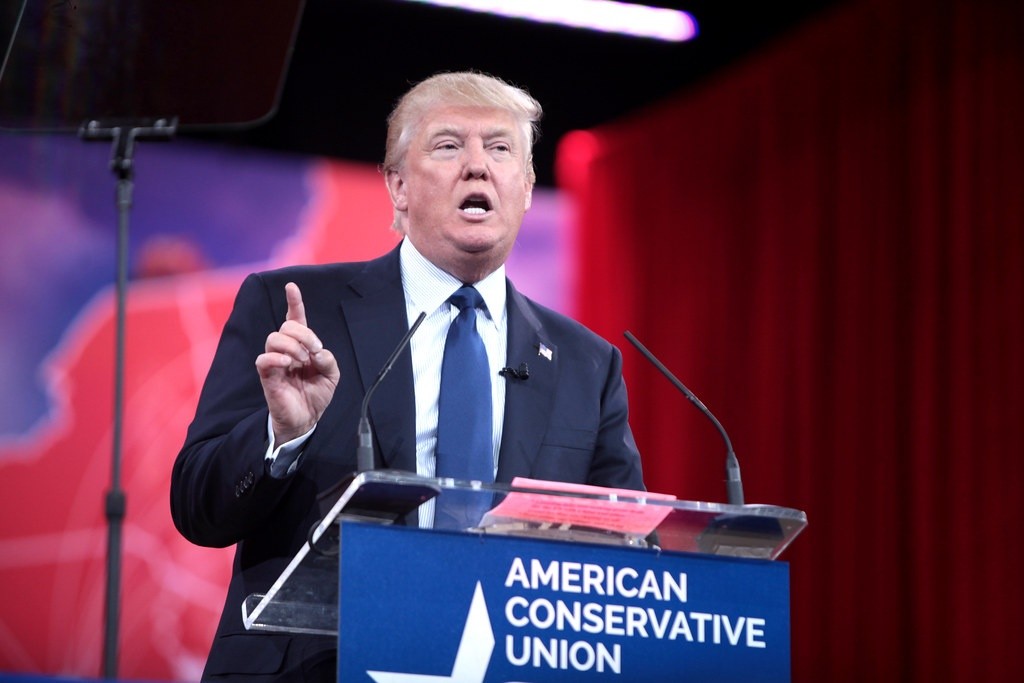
171, 73, 658, 683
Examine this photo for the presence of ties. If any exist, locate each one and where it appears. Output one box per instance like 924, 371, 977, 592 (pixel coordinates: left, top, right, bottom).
422, 285, 503, 529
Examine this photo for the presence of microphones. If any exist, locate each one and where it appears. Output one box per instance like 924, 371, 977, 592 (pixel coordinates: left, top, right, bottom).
622, 330, 785, 553
336, 312, 442, 516
499, 362, 529, 379
